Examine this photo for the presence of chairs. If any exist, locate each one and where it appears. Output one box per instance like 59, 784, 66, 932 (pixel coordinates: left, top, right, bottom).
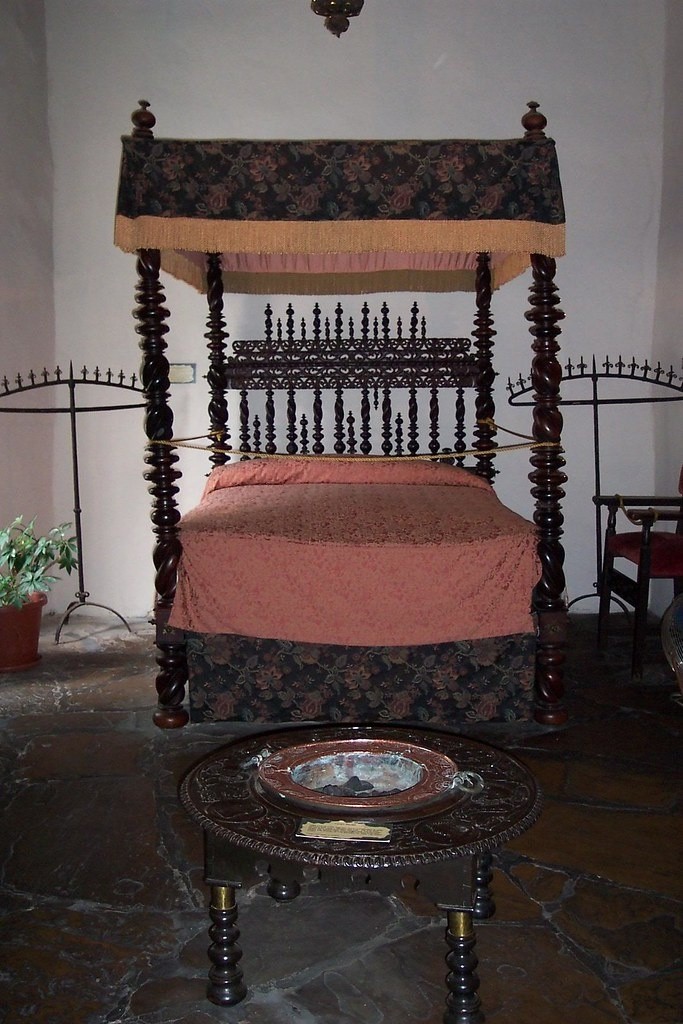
591, 464, 683, 680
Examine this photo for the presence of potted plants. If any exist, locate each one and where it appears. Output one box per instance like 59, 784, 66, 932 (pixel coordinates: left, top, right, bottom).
0, 515, 77, 672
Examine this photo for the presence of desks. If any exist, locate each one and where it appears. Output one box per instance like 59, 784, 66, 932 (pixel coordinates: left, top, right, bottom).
660, 596, 683, 694
179, 725, 542, 1024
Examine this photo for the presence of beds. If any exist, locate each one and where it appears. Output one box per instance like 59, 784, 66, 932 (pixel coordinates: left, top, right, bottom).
112, 97, 571, 728
